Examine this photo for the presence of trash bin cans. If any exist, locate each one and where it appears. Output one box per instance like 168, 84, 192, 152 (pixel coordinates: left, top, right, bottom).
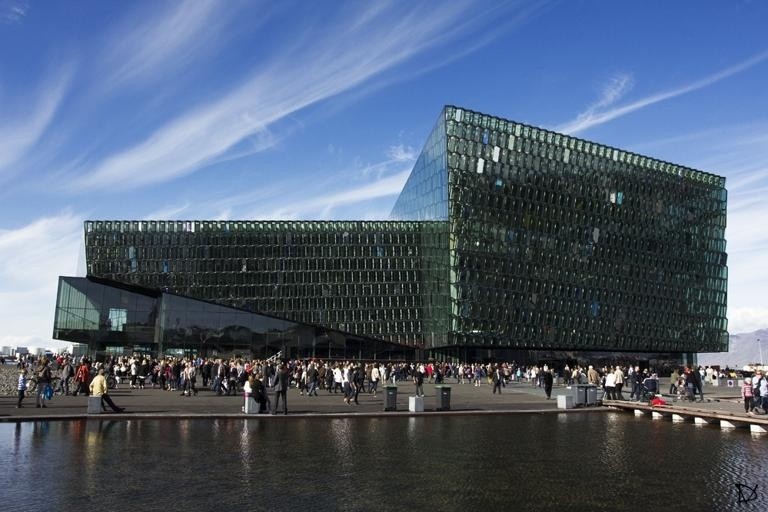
571, 384, 599, 408
434, 385, 451, 411
382, 385, 397, 411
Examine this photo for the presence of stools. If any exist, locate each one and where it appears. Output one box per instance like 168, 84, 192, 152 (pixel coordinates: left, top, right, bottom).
408, 396, 425, 411
244, 397, 259, 414
557, 394, 573, 410
87, 396, 103, 415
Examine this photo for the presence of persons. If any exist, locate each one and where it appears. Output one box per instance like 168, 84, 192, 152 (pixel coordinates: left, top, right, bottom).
0, 350, 768, 416
10, 418, 290, 486
